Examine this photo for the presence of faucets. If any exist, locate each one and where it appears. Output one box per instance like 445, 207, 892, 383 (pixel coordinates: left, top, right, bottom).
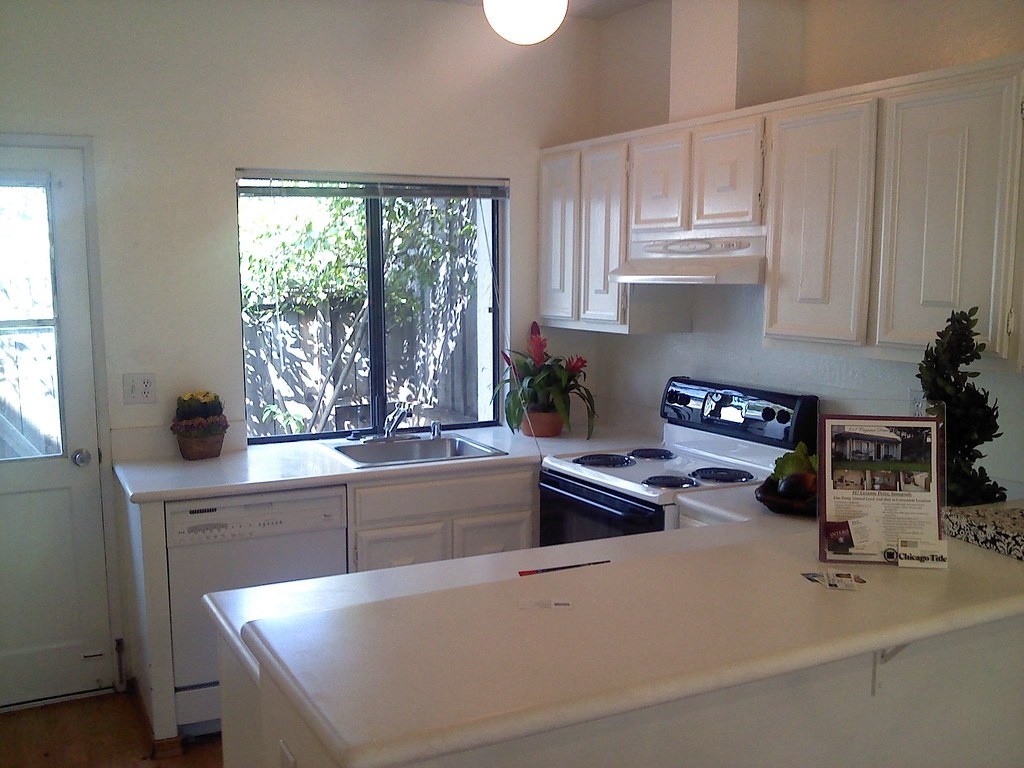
383, 402, 412, 438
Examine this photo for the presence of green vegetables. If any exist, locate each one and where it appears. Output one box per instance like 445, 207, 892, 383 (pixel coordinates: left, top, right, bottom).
771, 441, 817, 480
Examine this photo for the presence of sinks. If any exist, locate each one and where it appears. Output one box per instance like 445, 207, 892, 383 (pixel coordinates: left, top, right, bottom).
318, 432, 509, 469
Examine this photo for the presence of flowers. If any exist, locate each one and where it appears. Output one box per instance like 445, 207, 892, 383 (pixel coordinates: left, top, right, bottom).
488, 321, 598, 440
171, 391, 228, 439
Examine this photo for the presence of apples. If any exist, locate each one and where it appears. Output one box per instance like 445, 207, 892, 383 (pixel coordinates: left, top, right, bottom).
801, 471, 817, 492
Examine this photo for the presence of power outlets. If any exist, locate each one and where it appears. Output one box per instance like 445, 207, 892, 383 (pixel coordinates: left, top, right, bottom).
122, 372, 157, 407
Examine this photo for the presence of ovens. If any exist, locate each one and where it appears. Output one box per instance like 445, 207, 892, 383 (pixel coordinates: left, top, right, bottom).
538, 468, 679, 547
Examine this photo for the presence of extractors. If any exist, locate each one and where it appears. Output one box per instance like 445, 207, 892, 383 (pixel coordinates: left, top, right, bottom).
607, 236, 766, 285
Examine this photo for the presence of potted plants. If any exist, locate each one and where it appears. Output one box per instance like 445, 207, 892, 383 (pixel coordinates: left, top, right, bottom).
914, 306, 1008, 508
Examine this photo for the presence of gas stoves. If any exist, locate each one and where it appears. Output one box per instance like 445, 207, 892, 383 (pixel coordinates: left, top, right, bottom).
541, 376, 818, 506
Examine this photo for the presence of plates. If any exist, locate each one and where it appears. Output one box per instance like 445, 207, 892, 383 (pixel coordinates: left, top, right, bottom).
755, 488, 818, 516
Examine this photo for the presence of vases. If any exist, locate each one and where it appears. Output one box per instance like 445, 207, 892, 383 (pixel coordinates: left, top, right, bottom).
178, 435, 223, 461
522, 407, 563, 438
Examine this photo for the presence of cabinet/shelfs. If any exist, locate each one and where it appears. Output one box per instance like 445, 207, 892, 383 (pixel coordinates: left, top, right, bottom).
630, 104, 765, 241
538, 131, 691, 335
765, 51, 1024, 373
346, 461, 540, 575
